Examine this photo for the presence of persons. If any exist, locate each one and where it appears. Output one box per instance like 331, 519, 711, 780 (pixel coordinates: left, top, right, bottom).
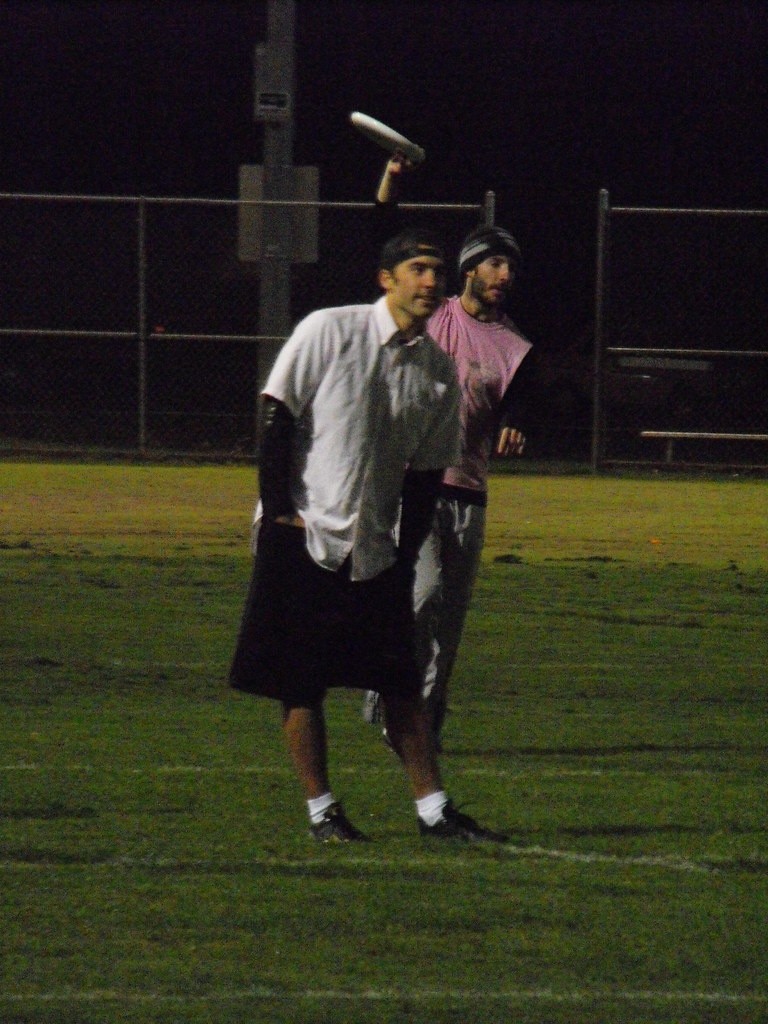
225, 153, 540, 843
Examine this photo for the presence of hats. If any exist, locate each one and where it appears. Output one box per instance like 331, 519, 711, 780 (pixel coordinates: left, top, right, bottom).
381, 229, 445, 269
458, 226, 521, 274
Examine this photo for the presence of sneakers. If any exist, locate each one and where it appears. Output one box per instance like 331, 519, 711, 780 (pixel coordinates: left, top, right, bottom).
419, 798, 509, 843
308, 803, 377, 846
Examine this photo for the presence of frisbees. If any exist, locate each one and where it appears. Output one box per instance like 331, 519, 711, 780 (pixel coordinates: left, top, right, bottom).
348, 111, 426, 162
